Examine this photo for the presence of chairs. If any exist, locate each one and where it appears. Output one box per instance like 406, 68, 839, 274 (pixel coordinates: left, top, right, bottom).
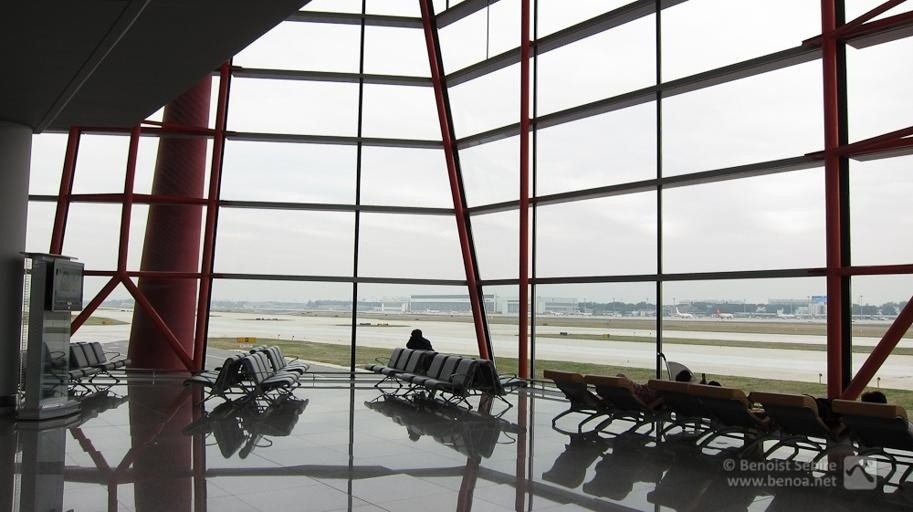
365, 348, 527, 409
542, 425, 913, 512
183, 345, 310, 407
543, 369, 913, 482
184, 395, 309, 458
364, 394, 527, 458
68, 390, 128, 425
21, 342, 132, 400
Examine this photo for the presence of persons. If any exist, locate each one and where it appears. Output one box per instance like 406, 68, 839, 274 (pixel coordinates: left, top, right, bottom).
616, 374, 662, 410
406, 329, 433, 350
676, 370, 691, 381
861, 391, 913, 432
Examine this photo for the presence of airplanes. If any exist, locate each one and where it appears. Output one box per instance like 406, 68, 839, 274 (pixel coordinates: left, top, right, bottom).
669, 306, 693, 319
713, 306, 735, 320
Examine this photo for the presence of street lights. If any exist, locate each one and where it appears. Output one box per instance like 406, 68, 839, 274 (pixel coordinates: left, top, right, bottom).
806, 295, 809, 318
742, 298, 745, 316
789, 298, 793, 312
859, 296, 862, 320
